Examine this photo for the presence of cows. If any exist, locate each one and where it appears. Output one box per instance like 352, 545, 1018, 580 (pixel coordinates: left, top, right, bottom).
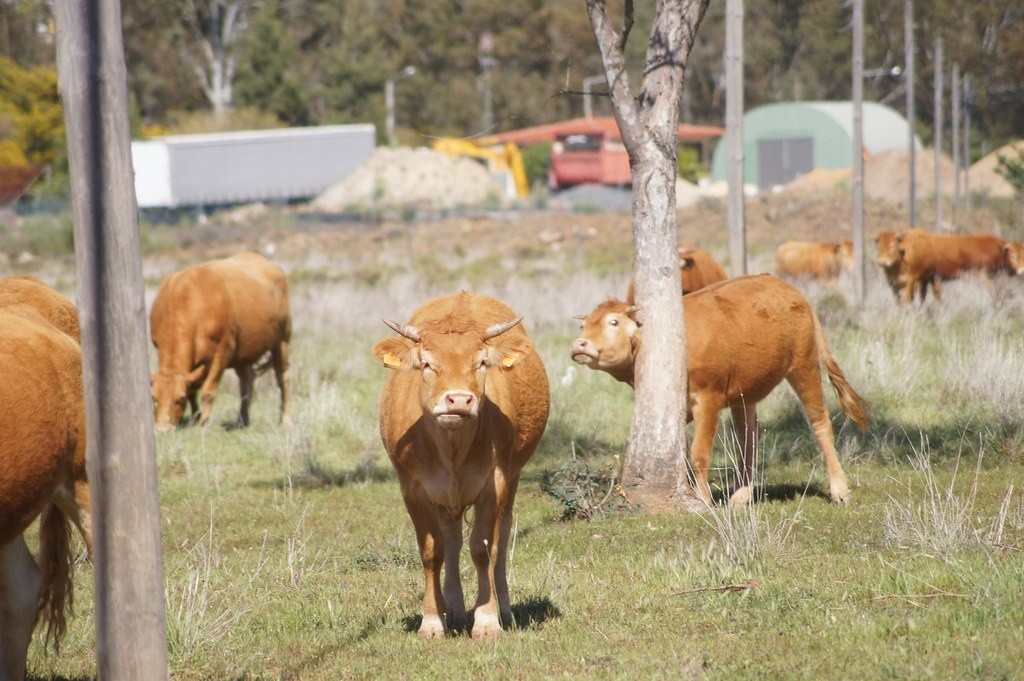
0, 277, 90, 681
372, 292, 551, 641
626, 245, 723, 308
875, 230, 942, 307
775, 240, 855, 281
148, 252, 292, 431
570, 274, 864, 508
899, 231, 1024, 304
